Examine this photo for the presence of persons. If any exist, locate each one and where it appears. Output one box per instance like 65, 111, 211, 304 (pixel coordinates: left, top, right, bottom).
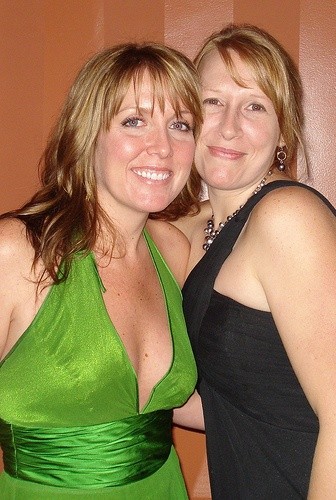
0, 42, 205, 500
165, 24, 336, 500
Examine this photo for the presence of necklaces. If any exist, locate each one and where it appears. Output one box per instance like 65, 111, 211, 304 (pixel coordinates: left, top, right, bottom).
203, 166, 272, 250
